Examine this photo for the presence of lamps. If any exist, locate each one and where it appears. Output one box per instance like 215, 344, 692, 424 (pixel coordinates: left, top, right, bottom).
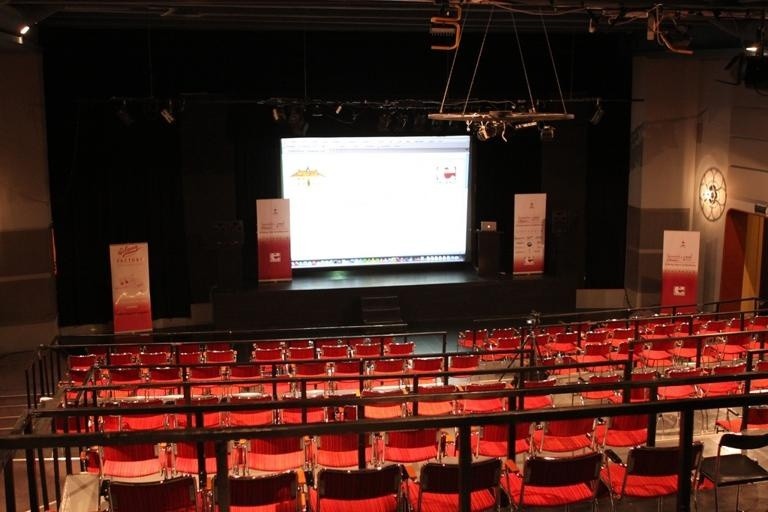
116, 93, 607, 136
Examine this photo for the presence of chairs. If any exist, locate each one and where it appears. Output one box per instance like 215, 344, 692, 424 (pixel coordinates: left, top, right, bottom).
48, 313, 767, 512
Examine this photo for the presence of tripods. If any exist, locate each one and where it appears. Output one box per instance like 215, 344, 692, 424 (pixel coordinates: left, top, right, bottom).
498, 334, 547, 382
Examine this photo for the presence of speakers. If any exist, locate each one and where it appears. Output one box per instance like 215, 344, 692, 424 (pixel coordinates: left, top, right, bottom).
479, 228, 512, 279
217, 248, 245, 293
